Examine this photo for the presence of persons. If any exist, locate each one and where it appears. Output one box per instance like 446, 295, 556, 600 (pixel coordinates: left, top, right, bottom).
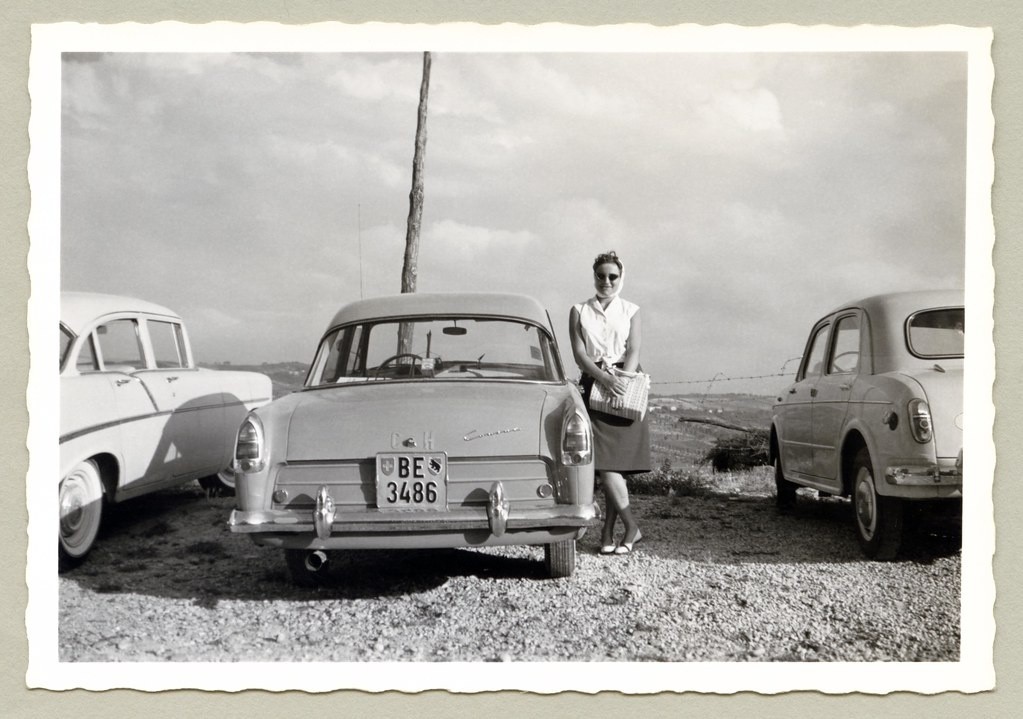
570, 250, 652, 556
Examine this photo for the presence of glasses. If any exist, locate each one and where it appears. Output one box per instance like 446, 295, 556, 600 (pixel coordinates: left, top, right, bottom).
595, 273, 618, 281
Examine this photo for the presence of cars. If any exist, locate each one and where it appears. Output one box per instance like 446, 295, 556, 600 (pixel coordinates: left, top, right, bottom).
57, 289, 275, 575
768, 289, 965, 563
226, 290, 604, 578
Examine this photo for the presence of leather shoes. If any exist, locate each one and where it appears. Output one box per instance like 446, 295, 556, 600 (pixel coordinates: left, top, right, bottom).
614, 528, 643, 555
601, 537, 616, 554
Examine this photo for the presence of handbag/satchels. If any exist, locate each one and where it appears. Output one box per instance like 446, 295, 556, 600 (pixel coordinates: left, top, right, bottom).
588, 365, 651, 422
